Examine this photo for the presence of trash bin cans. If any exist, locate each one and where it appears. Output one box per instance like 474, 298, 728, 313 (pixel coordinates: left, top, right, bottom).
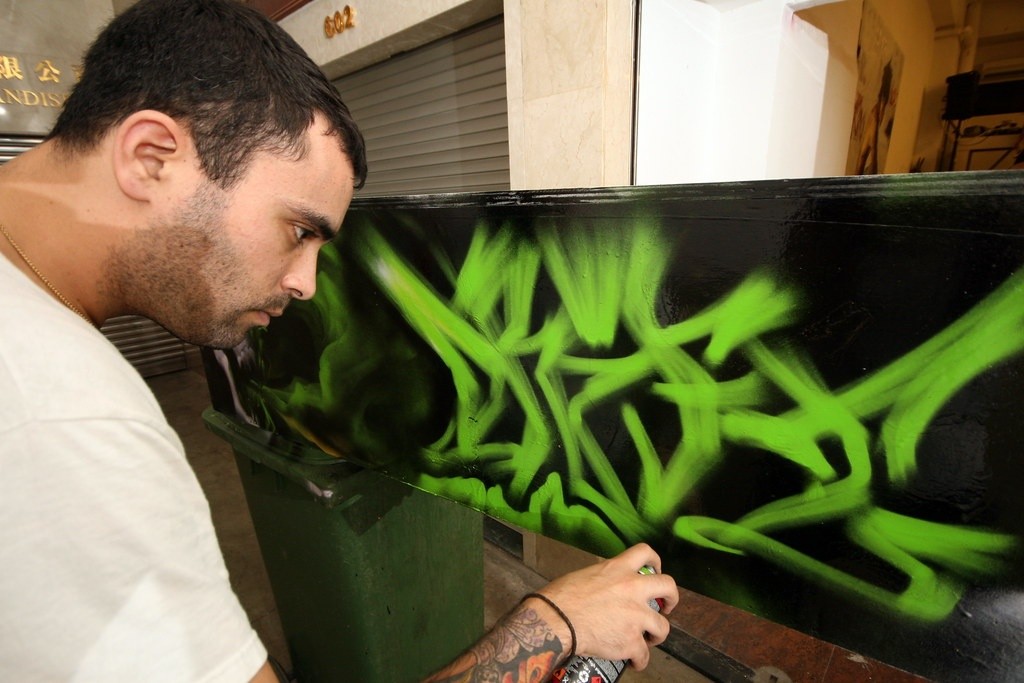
198, 405, 484, 683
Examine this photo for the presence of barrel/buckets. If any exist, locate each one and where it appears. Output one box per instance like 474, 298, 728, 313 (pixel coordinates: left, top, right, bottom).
199, 403, 485, 683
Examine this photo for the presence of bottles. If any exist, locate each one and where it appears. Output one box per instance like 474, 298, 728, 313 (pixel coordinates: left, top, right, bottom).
539, 564, 666, 683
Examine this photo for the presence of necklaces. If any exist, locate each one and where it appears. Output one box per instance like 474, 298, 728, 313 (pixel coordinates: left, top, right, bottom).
0, 224, 96, 328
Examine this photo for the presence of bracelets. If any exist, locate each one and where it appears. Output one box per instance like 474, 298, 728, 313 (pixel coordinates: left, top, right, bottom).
522, 593, 577, 667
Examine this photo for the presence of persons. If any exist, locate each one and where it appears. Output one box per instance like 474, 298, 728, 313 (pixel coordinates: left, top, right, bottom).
0, 0, 680, 683
854, 62, 894, 175
